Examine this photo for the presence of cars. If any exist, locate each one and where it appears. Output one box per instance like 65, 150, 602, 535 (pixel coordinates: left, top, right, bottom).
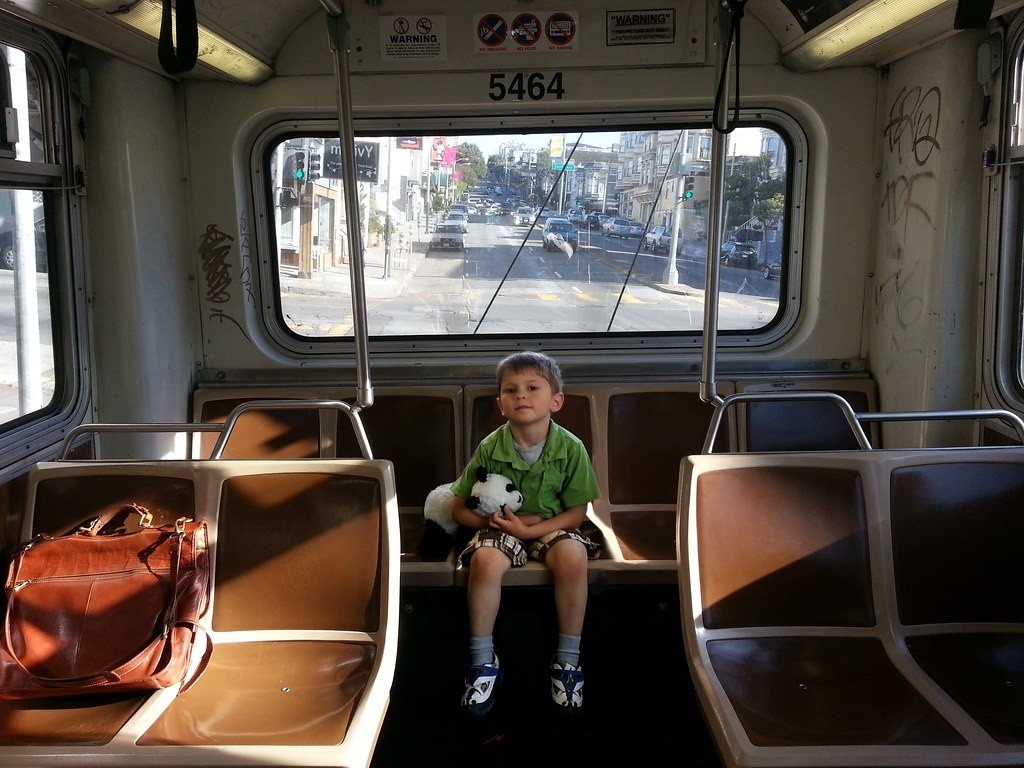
427, 179, 784, 280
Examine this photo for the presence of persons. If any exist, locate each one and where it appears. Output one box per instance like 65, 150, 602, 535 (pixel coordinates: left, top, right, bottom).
449, 350, 603, 727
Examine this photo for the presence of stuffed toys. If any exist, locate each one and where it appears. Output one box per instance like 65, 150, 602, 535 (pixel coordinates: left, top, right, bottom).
416, 465, 524, 563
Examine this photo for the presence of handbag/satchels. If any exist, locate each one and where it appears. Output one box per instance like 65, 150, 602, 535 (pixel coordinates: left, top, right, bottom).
0, 498, 213, 701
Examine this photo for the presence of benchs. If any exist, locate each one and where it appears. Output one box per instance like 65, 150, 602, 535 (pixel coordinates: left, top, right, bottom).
676, 391, 1024, 768
0, 401, 402, 768
183, 370, 887, 588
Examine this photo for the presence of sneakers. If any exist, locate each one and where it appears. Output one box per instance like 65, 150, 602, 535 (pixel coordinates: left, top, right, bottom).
461, 653, 500, 703
548, 654, 585, 707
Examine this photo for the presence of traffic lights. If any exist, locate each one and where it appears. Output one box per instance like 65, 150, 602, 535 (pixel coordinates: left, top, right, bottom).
296, 151, 304, 180
